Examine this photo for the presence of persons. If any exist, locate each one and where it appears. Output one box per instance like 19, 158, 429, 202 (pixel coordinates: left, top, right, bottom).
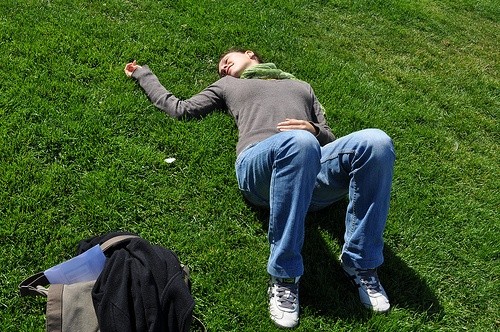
124, 46, 396, 328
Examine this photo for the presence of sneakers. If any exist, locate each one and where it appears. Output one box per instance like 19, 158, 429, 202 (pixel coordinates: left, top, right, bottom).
268, 275, 301, 328
341, 260, 390, 312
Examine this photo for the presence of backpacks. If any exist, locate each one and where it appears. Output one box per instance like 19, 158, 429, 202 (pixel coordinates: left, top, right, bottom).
19, 231, 207, 331
